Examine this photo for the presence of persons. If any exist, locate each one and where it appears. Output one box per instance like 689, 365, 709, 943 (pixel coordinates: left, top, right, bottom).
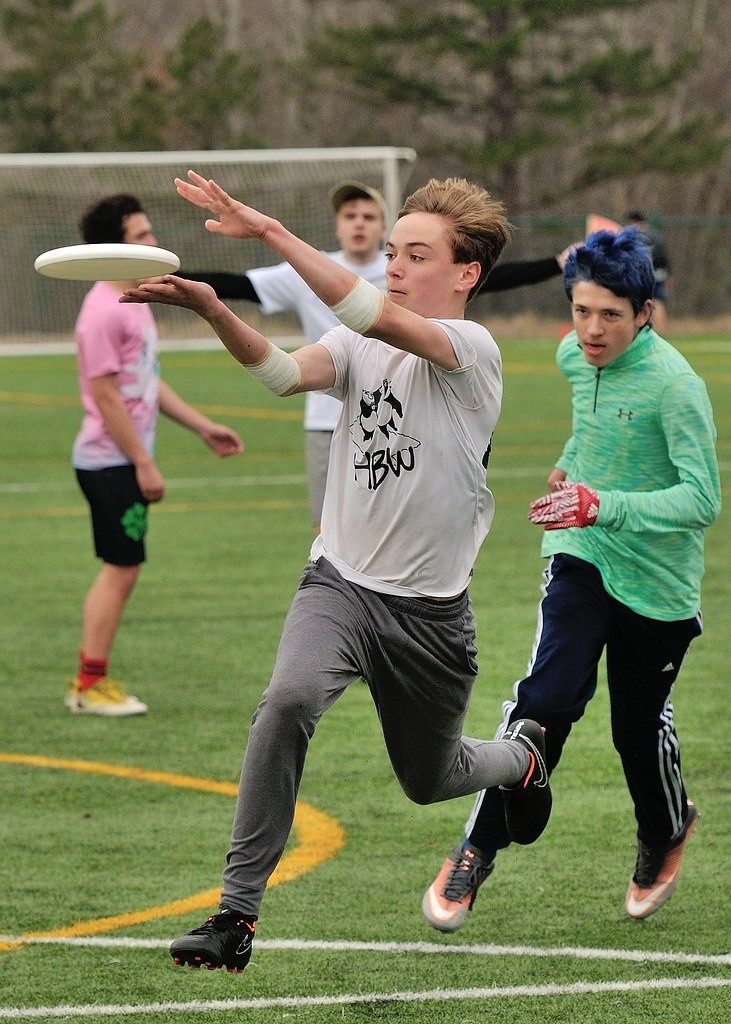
138, 177, 589, 537
64, 194, 243, 718
118, 169, 551, 974
423, 226, 720, 934
628, 214, 671, 329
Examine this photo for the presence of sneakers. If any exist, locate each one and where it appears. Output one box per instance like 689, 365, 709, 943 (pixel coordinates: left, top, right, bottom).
420, 843, 495, 935
500, 718, 553, 846
624, 797, 700, 920
62, 672, 139, 707
169, 908, 258, 974
72, 677, 150, 717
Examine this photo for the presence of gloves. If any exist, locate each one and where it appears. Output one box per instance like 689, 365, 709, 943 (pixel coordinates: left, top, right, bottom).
528, 480, 600, 531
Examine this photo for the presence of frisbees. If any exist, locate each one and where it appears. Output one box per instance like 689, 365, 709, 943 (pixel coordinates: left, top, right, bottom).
34, 243, 183, 282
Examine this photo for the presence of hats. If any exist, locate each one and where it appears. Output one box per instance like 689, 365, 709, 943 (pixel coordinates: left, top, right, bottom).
330, 177, 389, 217
